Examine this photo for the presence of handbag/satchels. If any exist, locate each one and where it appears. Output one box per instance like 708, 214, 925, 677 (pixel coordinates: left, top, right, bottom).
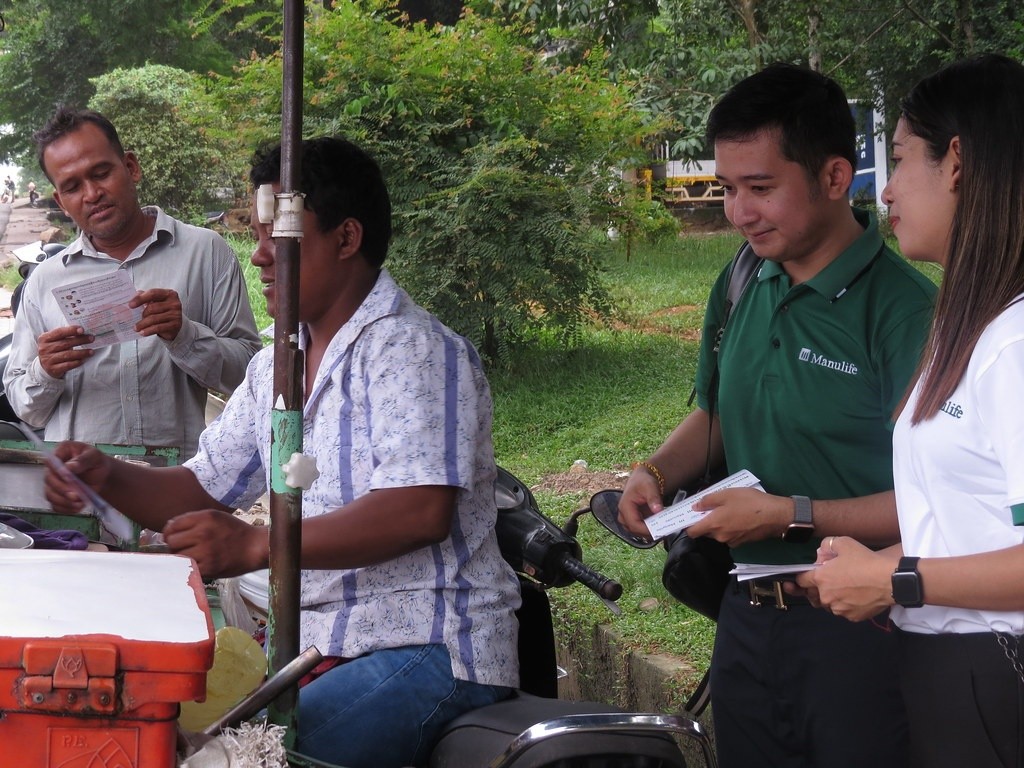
663, 478, 734, 621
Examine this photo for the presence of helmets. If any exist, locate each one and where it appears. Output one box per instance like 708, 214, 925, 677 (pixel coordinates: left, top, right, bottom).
11, 243, 66, 280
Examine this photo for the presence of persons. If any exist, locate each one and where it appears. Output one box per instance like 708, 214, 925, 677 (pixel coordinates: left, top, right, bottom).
616, 62, 939, 768
6, 175, 16, 203
29, 177, 37, 208
42, 139, 520, 768
782, 55, 1024, 768
2, 108, 263, 464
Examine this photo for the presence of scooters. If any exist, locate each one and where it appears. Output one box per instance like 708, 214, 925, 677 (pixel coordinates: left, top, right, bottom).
233, 463, 719, 768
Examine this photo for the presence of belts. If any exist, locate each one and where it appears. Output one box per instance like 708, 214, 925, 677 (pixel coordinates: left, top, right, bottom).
742, 575, 811, 612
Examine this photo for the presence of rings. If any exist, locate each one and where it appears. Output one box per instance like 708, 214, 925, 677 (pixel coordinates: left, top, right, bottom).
829, 536, 833, 553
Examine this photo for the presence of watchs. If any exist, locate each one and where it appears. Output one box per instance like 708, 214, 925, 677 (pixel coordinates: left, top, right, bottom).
890, 557, 923, 607
783, 495, 815, 543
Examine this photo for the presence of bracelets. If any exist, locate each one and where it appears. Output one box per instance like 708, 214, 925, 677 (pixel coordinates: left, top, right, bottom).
636, 462, 664, 495
